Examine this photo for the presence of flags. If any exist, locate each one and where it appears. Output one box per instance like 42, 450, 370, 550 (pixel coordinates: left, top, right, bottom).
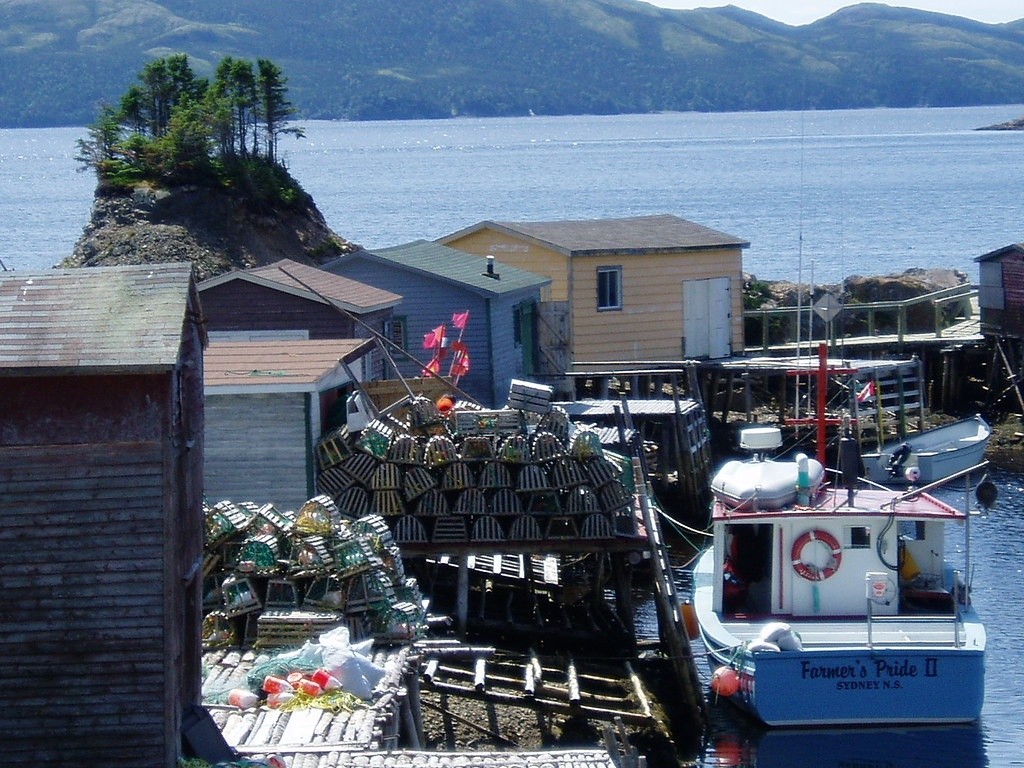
857, 379, 876, 402
424, 325, 446, 350
450, 340, 470, 377
421, 359, 441, 377
453, 313, 469, 330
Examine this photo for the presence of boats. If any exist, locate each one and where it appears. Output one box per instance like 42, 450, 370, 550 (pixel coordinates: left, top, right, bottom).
689, 233, 990, 726
859, 413, 989, 487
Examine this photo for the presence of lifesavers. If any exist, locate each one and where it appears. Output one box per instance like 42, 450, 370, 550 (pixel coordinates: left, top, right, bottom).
791, 529, 842, 584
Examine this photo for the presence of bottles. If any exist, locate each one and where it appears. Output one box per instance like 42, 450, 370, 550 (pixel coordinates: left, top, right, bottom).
262, 669, 343, 708
228, 688, 259, 709
